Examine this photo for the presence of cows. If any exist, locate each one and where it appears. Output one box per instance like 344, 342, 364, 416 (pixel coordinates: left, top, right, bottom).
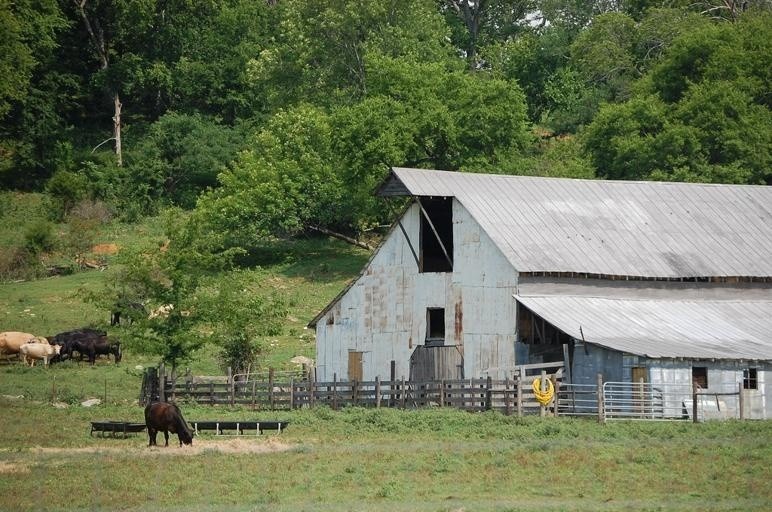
144, 401, 196, 447
0, 329, 122, 369
109, 303, 151, 326
